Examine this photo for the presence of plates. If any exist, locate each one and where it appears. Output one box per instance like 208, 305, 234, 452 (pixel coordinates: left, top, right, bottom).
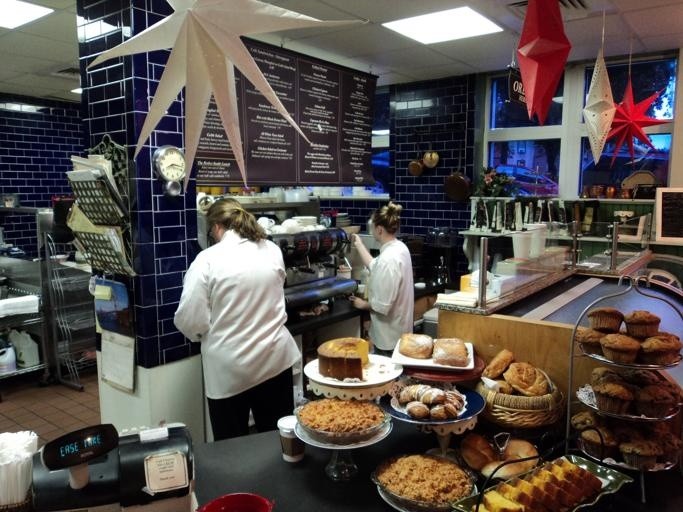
294, 420, 394, 450
377, 470, 477, 512
336, 213, 361, 234
379, 386, 487, 426
452, 454, 633, 512
292, 216, 318, 229
391, 338, 475, 371
304, 353, 404, 388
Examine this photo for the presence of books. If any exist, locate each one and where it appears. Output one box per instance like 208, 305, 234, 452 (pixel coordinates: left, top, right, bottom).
66, 155, 135, 396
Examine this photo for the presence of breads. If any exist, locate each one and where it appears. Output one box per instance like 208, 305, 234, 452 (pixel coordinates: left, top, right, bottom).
394, 383, 463, 420
479, 461, 618, 511
431, 339, 468, 367
399, 331, 432, 360
478, 350, 552, 399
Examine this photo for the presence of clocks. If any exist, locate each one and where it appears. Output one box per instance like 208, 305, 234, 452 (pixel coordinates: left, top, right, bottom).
152, 143, 188, 198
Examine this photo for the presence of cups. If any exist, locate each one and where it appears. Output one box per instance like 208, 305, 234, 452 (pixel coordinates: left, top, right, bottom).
276, 414, 306, 464
195, 492, 274, 512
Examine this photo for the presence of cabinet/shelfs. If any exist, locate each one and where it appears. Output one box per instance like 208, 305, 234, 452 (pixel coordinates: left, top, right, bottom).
458, 196, 683, 272
0, 206, 55, 387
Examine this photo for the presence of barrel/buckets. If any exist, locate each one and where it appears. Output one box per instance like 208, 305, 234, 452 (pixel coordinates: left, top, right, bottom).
0, 342, 16, 376
15, 331, 41, 368
7, 330, 19, 343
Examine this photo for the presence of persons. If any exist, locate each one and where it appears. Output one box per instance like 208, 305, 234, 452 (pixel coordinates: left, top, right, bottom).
173, 197, 303, 443
349, 201, 415, 359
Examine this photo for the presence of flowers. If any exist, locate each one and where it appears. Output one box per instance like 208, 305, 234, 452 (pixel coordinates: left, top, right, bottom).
473, 164, 517, 199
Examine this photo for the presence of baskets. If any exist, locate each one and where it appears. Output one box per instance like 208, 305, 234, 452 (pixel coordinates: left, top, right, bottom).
474, 367, 566, 430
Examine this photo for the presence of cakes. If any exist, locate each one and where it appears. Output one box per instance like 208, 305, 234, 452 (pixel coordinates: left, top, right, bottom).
376, 455, 471, 506
572, 309, 682, 471
317, 336, 370, 380
300, 399, 382, 433
